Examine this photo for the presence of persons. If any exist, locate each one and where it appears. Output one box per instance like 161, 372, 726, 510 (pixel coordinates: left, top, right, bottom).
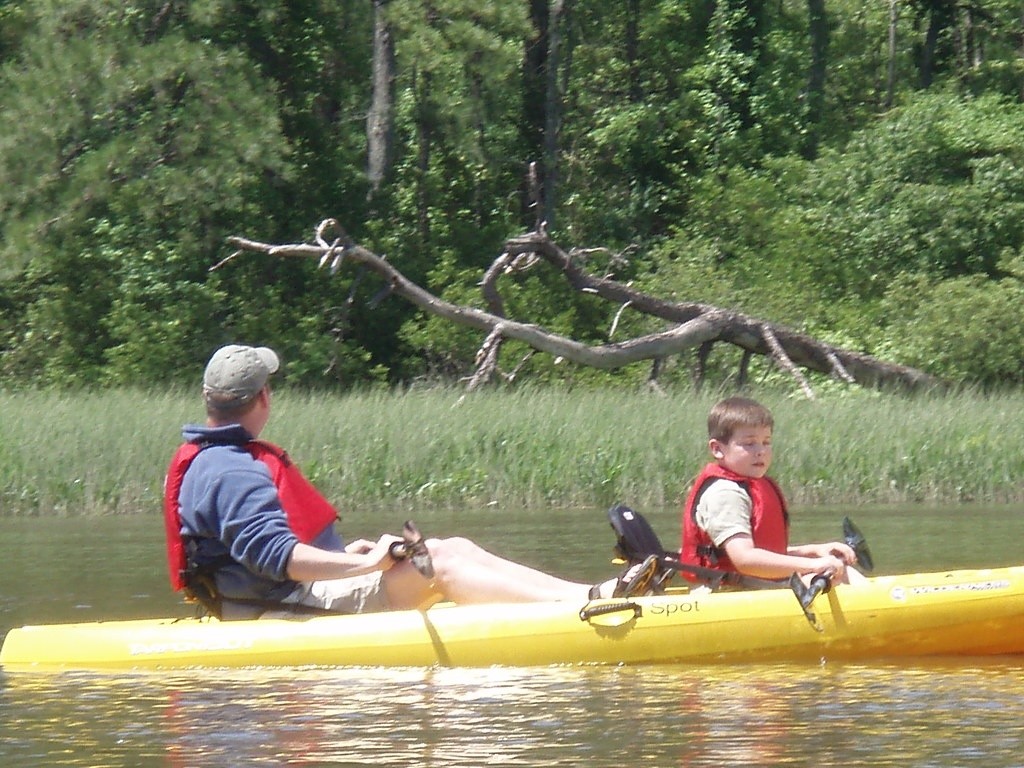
163, 344, 657, 617
679, 398, 868, 594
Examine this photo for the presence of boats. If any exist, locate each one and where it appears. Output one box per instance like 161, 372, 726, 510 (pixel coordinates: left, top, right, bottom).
0, 553, 1024, 667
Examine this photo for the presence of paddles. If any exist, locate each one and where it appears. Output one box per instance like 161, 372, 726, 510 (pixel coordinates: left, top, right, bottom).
786, 512, 875, 637
386, 517, 441, 584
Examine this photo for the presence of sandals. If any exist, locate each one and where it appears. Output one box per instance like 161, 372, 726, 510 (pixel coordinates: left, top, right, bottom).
591, 555, 660, 601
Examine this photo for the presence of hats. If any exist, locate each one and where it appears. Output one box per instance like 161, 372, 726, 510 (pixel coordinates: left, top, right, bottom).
203, 344, 280, 407
